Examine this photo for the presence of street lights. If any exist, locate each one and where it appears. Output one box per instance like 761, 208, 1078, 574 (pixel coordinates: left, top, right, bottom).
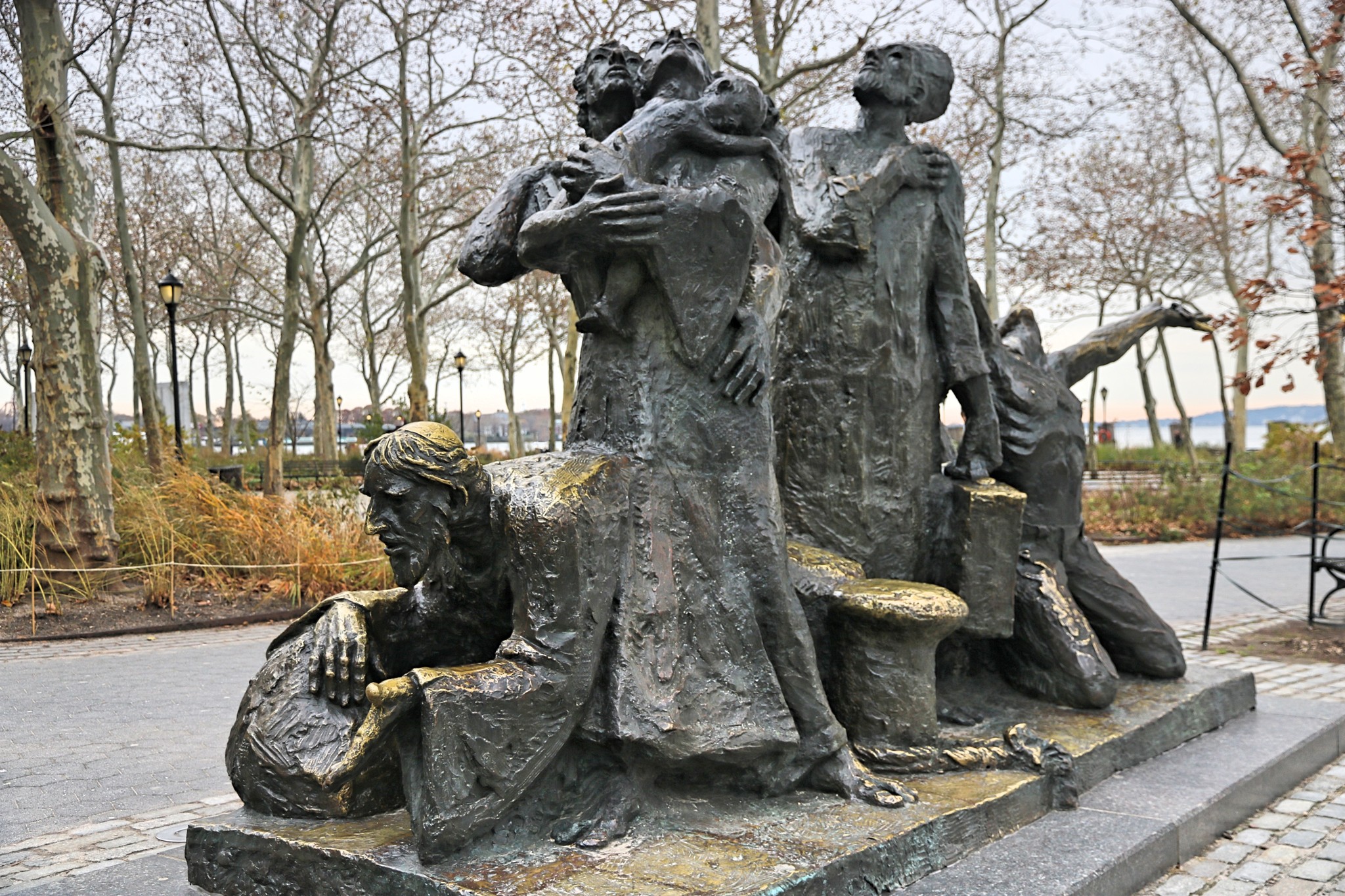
1100, 387, 1108, 444
455, 348, 467, 445
18, 336, 36, 436
474, 410, 482, 450
336, 396, 342, 454
367, 412, 372, 444
158, 268, 185, 473
396, 414, 401, 428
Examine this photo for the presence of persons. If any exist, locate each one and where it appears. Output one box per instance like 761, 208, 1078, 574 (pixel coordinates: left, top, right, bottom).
574, 73, 790, 343
263, 419, 630, 853
754, 43, 1000, 576
960, 256, 1213, 712
524, 20, 923, 853
462, 38, 778, 409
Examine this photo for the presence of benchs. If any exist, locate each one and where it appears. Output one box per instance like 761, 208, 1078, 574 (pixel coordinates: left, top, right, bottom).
258, 458, 367, 491
